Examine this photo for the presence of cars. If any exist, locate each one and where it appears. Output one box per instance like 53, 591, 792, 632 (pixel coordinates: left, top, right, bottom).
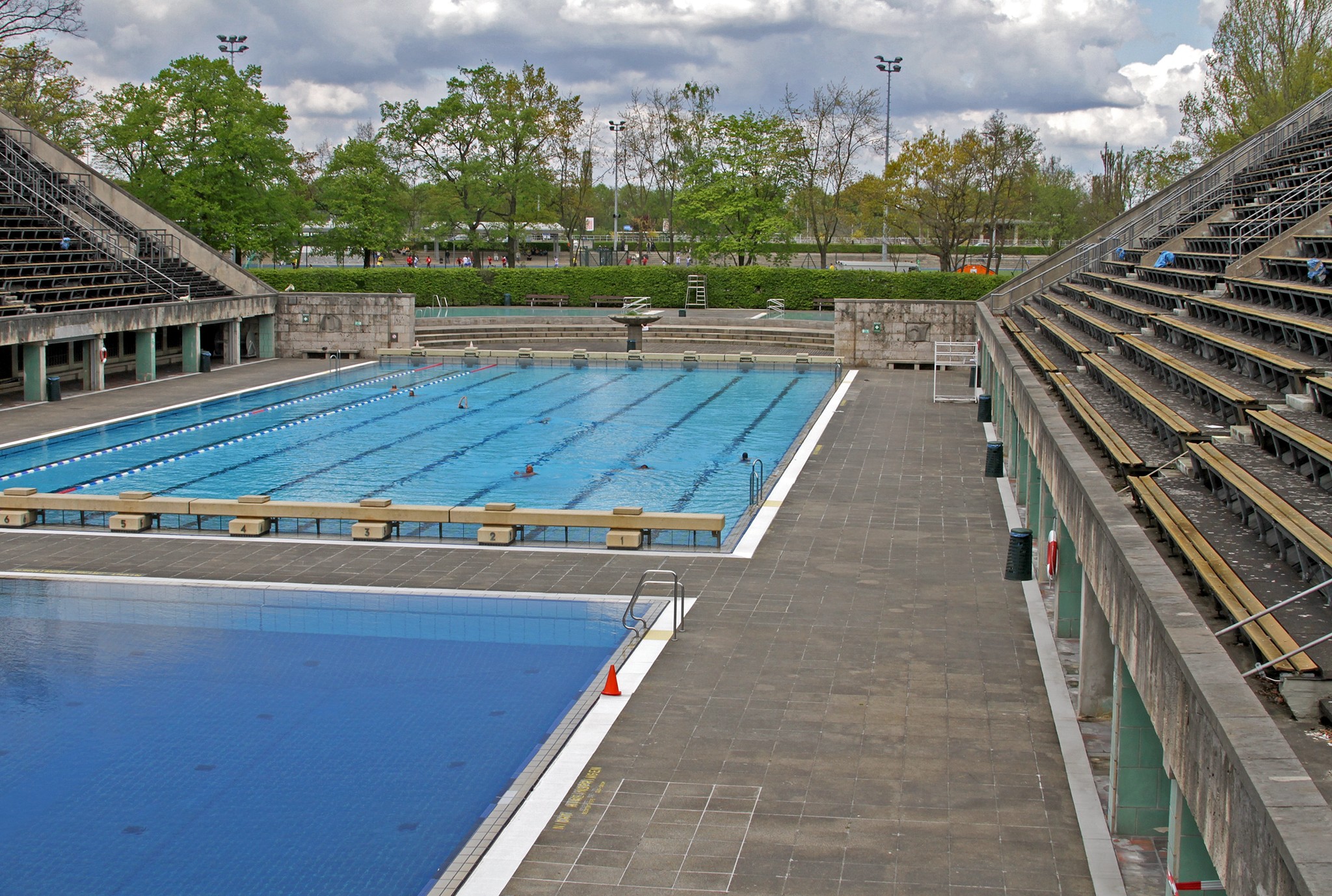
973, 242, 991, 246
451, 234, 468, 241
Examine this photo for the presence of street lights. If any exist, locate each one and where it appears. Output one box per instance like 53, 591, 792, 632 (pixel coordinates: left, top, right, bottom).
609, 120, 626, 251
874, 54, 902, 261
217, 34, 249, 266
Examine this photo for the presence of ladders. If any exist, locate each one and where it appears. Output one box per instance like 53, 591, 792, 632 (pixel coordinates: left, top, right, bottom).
684, 274, 708, 310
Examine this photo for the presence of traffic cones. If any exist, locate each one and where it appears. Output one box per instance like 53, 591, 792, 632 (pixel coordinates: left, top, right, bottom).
601, 664, 621, 696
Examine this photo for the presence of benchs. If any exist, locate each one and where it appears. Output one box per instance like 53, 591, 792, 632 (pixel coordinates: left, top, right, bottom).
0, 130, 233, 317
1000, 119, 1332, 686
525, 294, 569, 307
589, 295, 647, 308
813, 298, 835, 312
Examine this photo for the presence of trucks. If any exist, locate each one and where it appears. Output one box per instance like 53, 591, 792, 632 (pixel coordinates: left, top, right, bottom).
835, 260, 921, 273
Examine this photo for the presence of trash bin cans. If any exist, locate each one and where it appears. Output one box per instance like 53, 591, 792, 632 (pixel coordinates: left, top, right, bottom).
200, 351, 212, 372
909, 267, 919, 271
47, 376, 62, 401
504, 293, 511, 306
977, 394, 991, 423
968, 366, 980, 387
626, 339, 636, 353
678, 310, 686, 317
1004, 528, 1034, 581
985, 441, 1003, 477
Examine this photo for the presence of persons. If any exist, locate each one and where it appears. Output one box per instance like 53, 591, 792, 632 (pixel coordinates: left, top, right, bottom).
458, 396, 468, 408
826, 263, 835, 270
641, 465, 648, 468
291, 245, 695, 267
905, 257, 921, 273
409, 391, 417, 397
515, 465, 536, 475
740, 452, 750, 460
390, 385, 398, 391
